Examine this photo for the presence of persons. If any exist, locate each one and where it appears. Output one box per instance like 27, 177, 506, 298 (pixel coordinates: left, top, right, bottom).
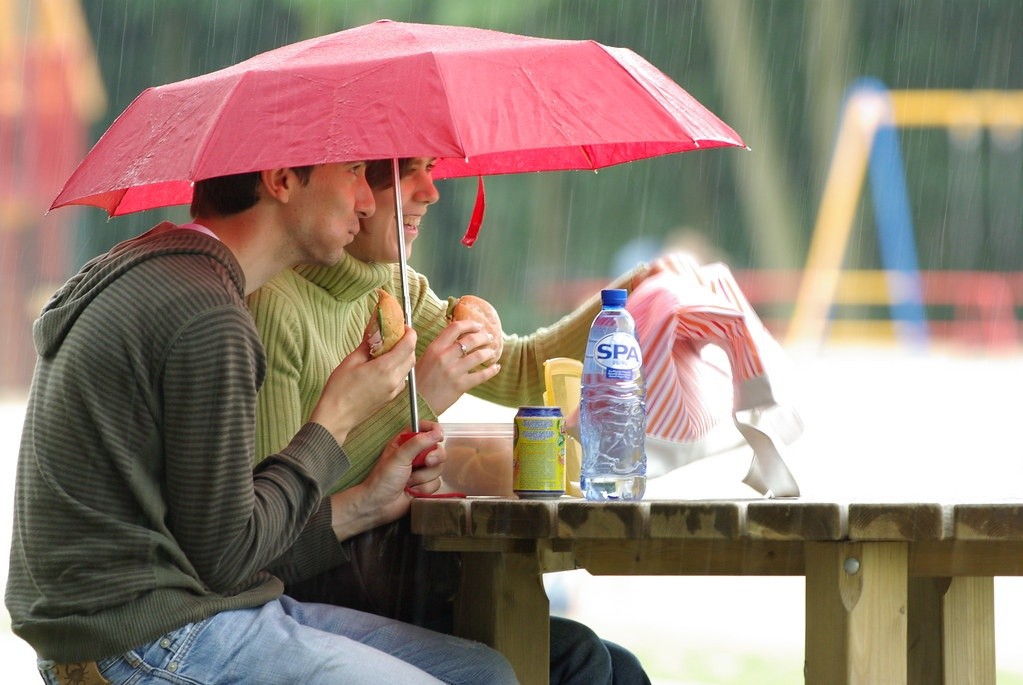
5, 157, 706, 685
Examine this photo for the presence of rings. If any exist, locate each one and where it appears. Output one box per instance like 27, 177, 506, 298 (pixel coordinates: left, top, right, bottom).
456, 341, 467, 358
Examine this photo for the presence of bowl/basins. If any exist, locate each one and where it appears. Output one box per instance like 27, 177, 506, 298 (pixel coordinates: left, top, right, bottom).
437, 423, 515, 497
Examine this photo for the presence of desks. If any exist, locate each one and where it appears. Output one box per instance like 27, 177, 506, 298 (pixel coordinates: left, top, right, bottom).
411, 495, 1023, 685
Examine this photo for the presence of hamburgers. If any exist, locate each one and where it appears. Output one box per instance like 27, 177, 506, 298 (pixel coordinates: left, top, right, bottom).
446, 294, 503, 368
362, 288, 404, 358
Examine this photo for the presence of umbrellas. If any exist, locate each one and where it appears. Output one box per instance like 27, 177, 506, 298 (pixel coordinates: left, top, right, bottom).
45, 19, 753, 499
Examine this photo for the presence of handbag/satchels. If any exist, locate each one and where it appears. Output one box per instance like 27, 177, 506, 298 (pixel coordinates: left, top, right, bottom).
589, 259, 800, 501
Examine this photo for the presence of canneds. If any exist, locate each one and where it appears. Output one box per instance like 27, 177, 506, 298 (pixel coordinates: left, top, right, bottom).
512, 405, 568, 500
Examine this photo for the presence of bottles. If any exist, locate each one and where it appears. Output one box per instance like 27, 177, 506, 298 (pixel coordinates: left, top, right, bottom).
580, 289, 647, 502
